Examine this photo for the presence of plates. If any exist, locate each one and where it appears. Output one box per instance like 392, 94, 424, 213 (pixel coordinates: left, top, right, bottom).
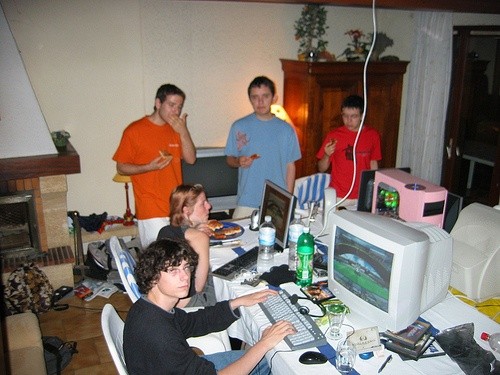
210, 222, 244, 240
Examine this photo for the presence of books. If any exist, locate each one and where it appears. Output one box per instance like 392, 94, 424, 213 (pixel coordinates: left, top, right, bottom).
74, 278, 119, 301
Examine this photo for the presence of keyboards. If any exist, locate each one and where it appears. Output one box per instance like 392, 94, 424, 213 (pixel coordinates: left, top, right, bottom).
258, 289, 327, 350
212, 246, 259, 281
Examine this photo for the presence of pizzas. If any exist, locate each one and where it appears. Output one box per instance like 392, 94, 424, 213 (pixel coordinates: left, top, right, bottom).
330, 138, 337, 145
250, 154, 260, 159
159, 150, 172, 158
208, 218, 242, 239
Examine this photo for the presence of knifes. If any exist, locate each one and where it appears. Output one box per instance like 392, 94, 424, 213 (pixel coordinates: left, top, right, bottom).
208, 240, 234, 245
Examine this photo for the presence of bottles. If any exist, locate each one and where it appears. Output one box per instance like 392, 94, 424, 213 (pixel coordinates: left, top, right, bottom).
481, 332, 500, 354
257, 216, 276, 274
288, 213, 305, 270
297, 227, 314, 287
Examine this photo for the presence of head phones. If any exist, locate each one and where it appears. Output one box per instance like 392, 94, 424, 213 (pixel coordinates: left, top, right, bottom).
288, 294, 326, 317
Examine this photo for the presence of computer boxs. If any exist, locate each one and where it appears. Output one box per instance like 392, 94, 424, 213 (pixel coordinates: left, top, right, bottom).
370, 168, 448, 231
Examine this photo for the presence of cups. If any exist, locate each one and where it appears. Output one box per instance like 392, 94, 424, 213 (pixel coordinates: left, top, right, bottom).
335, 342, 357, 374
326, 304, 347, 340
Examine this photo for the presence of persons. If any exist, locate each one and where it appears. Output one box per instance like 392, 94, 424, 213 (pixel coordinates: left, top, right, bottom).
123, 239, 298, 375
112, 84, 196, 249
316, 94, 382, 209
224, 76, 302, 218
157, 184, 243, 351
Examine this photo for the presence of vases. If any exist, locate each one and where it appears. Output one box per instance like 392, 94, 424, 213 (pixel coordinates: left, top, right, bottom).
347, 50, 366, 62
304, 51, 328, 64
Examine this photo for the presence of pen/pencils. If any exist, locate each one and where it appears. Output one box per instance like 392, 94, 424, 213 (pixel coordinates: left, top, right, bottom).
378, 355, 392, 373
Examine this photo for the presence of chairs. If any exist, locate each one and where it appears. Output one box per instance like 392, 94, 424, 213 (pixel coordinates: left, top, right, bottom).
101, 236, 143, 375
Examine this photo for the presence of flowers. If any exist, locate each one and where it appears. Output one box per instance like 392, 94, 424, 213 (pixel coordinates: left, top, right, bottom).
294, 4, 329, 54
337, 29, 369, 60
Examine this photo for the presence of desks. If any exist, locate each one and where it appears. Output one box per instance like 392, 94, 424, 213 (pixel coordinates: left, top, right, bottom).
209, 213, 500, 375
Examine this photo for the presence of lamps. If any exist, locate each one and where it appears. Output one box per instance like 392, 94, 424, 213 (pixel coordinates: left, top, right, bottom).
112, 173, 135, 227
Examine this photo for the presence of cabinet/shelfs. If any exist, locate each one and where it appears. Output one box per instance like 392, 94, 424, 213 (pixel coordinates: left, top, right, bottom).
278, 57, 409, 182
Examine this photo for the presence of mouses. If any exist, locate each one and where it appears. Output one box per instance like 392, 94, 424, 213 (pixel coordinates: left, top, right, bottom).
299, 351, 327, 364
250, 210, 260, 229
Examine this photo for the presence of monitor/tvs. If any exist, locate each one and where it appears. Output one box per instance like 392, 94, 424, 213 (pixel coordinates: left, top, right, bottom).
358, 167, 411, 214
180, 147, 239, 211
448, 202, 500, 302
328, 209, 453, 333
257, 179, 296, 252
